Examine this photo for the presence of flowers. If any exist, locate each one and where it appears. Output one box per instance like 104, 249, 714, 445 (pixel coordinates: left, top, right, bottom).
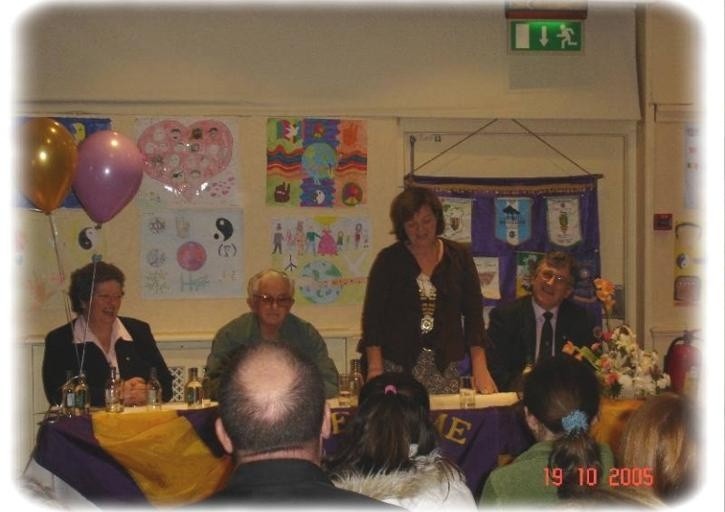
561, 277, 673, 399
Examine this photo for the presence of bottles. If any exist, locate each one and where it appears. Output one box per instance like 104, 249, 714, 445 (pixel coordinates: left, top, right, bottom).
184, 367, 203, 409
348, 359, 366, 405
147, 366, 162, 409
62, 370, 74, 415
74, 373, 90, 415
105, 366, 119, 412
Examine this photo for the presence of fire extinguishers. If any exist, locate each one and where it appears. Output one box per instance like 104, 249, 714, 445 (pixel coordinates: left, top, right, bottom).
663, 330, 705, 397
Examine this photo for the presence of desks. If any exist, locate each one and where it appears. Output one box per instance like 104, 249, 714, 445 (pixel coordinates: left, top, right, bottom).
20, 390, 648, 506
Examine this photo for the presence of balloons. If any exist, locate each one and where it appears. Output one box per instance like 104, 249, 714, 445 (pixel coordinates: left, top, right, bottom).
72, 129, 144, 377
15, 117, 81, 377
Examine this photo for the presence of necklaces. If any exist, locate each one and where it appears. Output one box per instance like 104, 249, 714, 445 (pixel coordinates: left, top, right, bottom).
407, 238, 442, 333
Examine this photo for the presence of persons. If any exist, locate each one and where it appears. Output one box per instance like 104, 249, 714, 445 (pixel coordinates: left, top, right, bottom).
478, 355, 627, 506
203, 270, 340, 401
42, 261, 173, 408
321, 371, 477, 509
186, 337, 399, 509
614, 390, 701, 504
355, 185, 499, 395
486, 249, 605, 397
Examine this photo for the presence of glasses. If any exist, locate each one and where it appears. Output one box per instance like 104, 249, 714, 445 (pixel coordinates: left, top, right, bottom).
253, 294, 292, 306
539, 270, 569, 288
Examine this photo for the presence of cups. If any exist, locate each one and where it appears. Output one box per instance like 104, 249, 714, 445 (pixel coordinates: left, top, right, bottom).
458, 375, 476, 409
338, 374, 354, 404
116, 379, 125, 412
